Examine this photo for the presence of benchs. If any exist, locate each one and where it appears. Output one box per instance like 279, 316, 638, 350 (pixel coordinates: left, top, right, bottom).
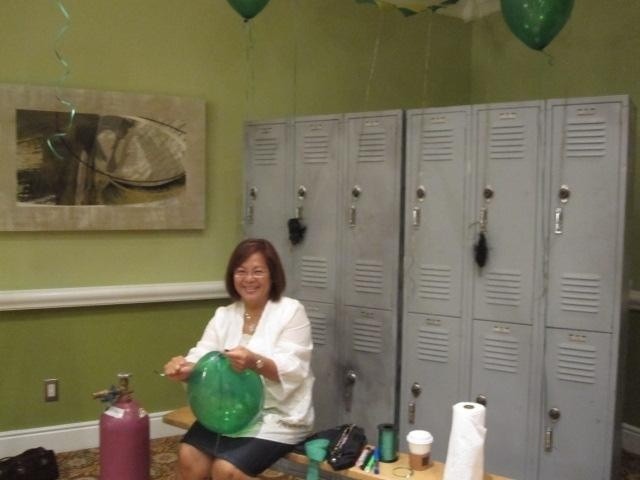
161, 404, 517, 480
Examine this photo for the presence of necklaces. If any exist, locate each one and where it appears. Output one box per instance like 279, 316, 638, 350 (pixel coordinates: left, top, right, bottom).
244, 311, 258, 333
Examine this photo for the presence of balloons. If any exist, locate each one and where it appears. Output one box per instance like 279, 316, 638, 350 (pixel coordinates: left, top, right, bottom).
500, 0, 574, 49
187, 350, 264, 433
228, 0, 270, 21
357, 0, 458, 17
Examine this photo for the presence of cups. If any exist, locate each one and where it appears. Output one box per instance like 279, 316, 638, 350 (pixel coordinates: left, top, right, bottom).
406, 431, 434, 472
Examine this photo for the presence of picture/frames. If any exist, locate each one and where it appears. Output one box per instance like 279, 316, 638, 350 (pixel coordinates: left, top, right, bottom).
0, 82, 207, 232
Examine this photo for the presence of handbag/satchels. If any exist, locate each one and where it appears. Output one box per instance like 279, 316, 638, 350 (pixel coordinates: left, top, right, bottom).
1, 446, 60, 479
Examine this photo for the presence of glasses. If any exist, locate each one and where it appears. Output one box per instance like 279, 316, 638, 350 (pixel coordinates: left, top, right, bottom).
233, 267, 268, 278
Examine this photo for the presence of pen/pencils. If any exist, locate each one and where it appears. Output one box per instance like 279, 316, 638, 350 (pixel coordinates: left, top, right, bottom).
357, 446, 379, 474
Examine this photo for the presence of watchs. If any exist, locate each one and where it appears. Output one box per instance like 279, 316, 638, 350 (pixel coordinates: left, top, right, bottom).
255, 355, 264, 373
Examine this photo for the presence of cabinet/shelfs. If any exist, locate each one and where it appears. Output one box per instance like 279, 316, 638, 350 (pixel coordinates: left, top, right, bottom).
241, 94, 636, 480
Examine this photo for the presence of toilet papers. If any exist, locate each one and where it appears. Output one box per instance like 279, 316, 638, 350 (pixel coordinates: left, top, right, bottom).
442, 401, 488, 480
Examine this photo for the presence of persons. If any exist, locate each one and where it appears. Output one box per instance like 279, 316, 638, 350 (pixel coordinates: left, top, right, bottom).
163, 239, 315, 480
